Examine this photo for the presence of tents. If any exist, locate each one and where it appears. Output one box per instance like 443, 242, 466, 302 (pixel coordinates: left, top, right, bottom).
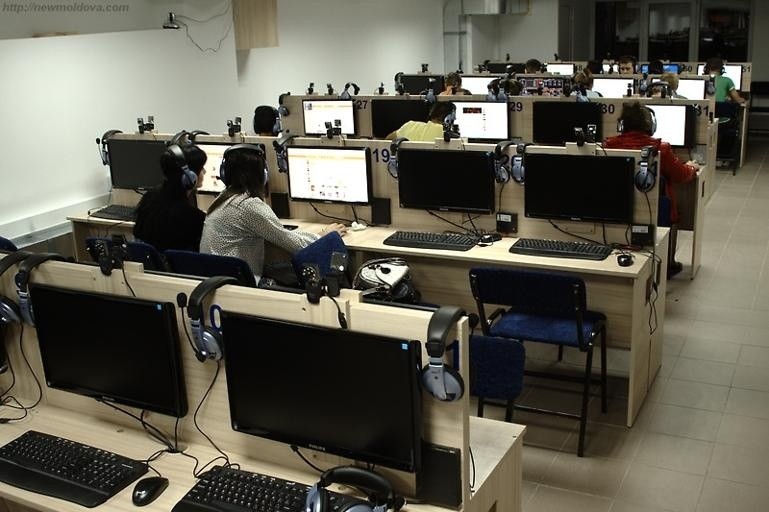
0, 250, 34, 327
19, 251, 74, 327
305, 466, 407, 511
176, 274, 238, 364
421, 305, 479, 404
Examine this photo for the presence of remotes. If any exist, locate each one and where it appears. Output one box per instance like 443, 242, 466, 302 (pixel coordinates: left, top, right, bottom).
618, 254, 633, 266
132, 476, 168, 506
481, 234, 494, 243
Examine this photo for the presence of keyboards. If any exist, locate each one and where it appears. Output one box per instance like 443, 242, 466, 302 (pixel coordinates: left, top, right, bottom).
0, 430, 149, 508
509, 237, 612, 260
169, 463, 374, 512
93, 204, 138, 222
383, 231, 479, 251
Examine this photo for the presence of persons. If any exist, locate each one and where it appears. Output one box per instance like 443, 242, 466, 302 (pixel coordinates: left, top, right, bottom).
600, 101, 701, 274
198, 145, 350, 289
383, 101, 456, 141
131, 143, 208, 255
253, 105, 280, 137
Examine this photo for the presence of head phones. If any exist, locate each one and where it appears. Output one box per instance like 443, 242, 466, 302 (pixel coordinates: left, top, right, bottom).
421, 305, 479, 404
305, 466, 407, 511
0, 250, 34, 327
19, 251, 74, 327
176, 274, 238, 364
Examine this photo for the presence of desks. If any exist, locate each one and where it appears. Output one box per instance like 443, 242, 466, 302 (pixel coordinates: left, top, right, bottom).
0, 57, 754, 512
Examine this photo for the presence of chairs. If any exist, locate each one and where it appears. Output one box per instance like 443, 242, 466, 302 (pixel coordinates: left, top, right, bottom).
717, 160, 730, 167
668, 262, 682, 276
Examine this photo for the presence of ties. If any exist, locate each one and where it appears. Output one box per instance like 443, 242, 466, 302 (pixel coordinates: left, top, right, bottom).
27, 281, 190, 419
217, 308, 424, 475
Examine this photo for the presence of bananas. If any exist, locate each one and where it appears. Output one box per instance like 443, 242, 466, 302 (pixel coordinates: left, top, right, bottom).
383, 231, 479, 251
0, 430, 149, 508
509, 237, 612, 260
169, 463, 374, 512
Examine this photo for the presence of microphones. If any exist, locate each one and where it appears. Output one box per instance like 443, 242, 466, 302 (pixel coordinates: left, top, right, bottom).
288, 441, 326, 474
176, 292, 204, 361
331, 292, 349, 330
465, 311, 479, 336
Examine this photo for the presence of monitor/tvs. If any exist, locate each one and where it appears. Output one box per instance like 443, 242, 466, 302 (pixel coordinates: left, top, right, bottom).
623, 102, 693, 149
399, 75, 446, 94
517, 76, 565, 96
398, 147, 496, 216
651, 77, 706, 100
532, 101, 603, 146
107, 139, 166, 190
460, 75, 502, 95
546, 62, 575, 76
523, 152, 635, 224
641, 64, 680, 76
302, 99, 356, 135
27, 281, 190, 419
697, 64, 743, 91
285, 144, 374, 206
370, 98, 429, 139
603, 63, 639, 75
192, 140, 266, 194
448, 99, 511, 143
487, 63, 523, 73
591, 77, 635, 98
217, 308, 424, 475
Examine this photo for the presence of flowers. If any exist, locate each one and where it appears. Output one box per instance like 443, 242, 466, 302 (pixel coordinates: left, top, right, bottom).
176, 292, 204, 361
331, 292, 349, 330
465, 311, 479, 336
288, 441, 326, 474
